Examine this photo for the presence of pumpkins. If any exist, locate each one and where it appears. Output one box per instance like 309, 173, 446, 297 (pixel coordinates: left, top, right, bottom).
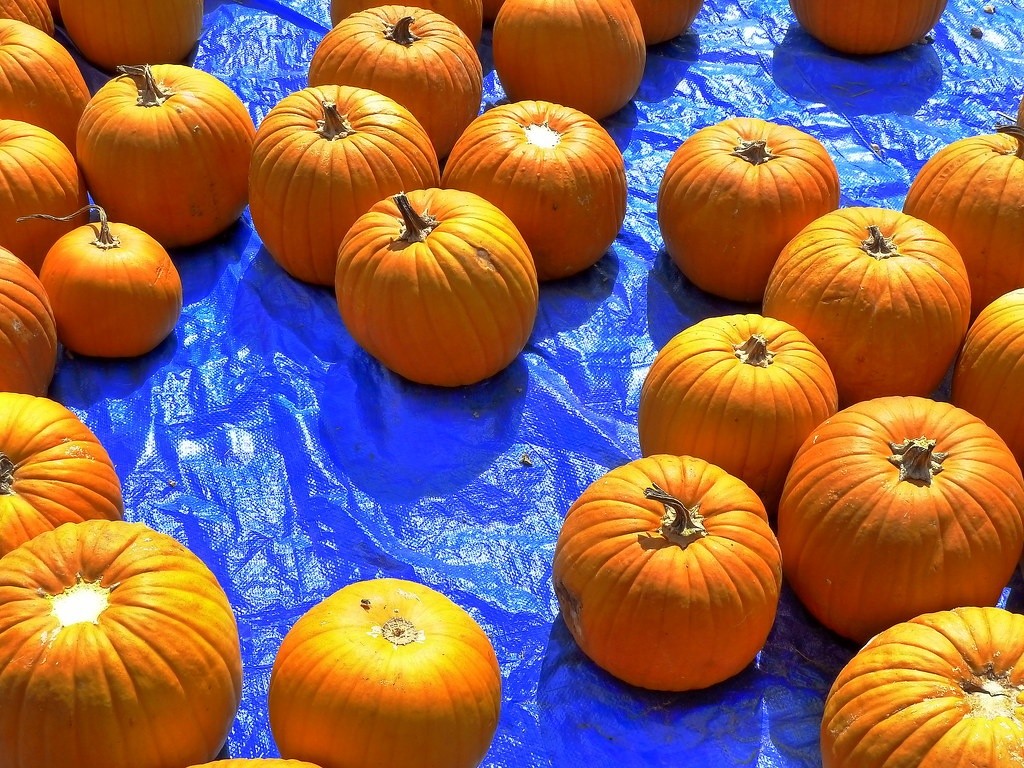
0, 0, 1024, 768
